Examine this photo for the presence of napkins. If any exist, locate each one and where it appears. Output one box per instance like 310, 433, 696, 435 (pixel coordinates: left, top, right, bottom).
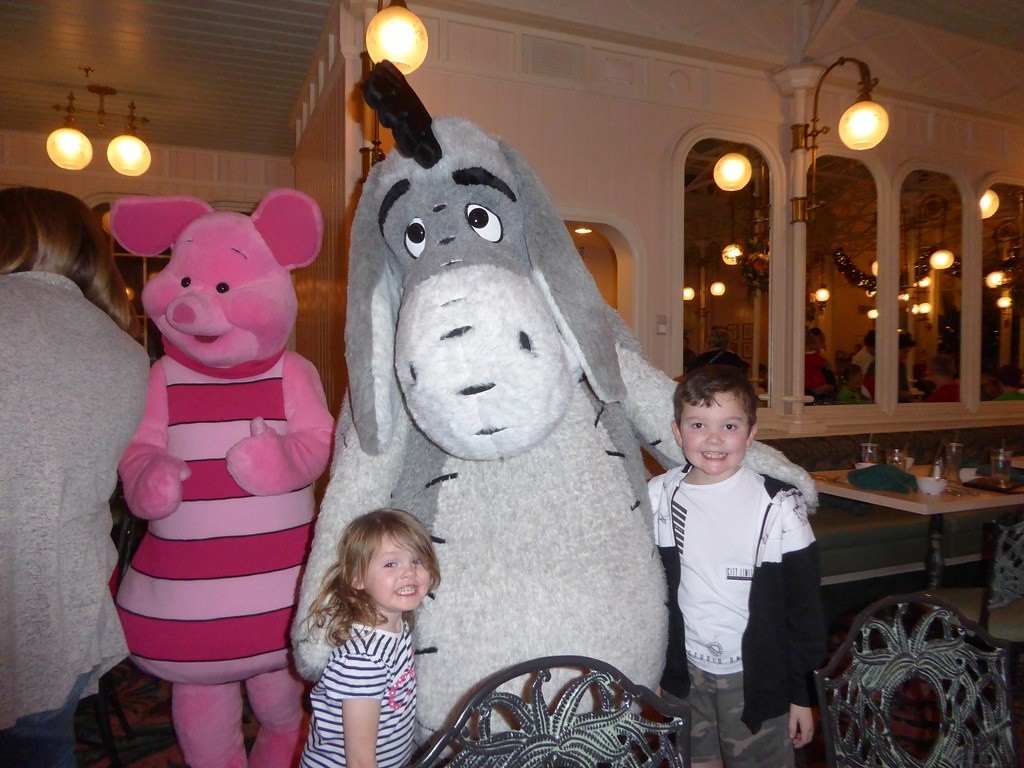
847, 464, 918, 494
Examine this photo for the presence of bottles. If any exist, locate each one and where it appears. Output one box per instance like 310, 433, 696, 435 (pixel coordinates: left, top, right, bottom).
931, 459, 944, 480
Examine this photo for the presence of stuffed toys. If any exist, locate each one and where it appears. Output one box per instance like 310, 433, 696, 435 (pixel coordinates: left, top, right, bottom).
102, 188, 341, 768
289, 61, 819, 767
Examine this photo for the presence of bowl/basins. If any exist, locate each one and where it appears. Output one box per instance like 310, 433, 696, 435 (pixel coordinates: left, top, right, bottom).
916, 476, 954, 495
854, 463, 878, 471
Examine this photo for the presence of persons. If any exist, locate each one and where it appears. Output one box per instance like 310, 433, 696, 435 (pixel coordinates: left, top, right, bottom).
294, 507, 442, 768
678, 325, 1024, 404
647, 348, 818, 768
1, 187, 151, 767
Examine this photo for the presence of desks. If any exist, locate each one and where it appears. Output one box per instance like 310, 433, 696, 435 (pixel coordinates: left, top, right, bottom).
810, 456, 1024, 591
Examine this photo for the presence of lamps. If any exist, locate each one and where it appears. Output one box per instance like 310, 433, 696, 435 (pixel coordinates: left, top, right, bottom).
365, 0, 428, 75
46, 86, 151, 177
804, 57, 890, 219
684, 153, 1010, 321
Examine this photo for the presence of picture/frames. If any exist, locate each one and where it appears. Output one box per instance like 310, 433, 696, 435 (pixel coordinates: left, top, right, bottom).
743, 322, 753, 339
742, 342, 752, 358
728, 323, 739, 340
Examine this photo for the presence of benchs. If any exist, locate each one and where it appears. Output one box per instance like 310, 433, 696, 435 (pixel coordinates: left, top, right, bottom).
756, 424, 1024, 586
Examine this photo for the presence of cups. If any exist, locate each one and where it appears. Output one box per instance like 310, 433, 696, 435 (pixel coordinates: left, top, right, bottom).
860, 443, 878, 463
886, 447, 906, 472
906, 457, 914, 469
991, 447, 1014, 484
946, 442, 964, 481
959, 467, 978, 483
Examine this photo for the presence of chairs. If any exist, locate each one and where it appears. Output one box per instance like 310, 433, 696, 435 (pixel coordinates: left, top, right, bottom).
410, 655, 690, 768
813, 592, 1017, 768
